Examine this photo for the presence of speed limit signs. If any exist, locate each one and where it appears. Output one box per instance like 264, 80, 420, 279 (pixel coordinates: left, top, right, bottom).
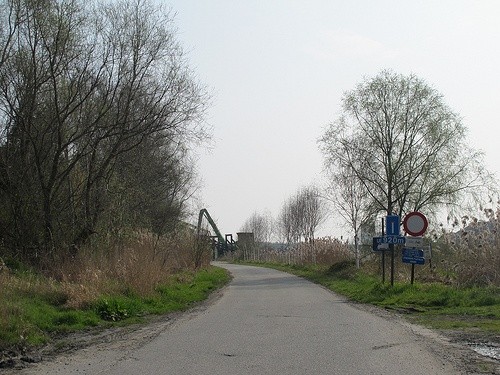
382, 236, 406, 244
373, 237, 390, 251
